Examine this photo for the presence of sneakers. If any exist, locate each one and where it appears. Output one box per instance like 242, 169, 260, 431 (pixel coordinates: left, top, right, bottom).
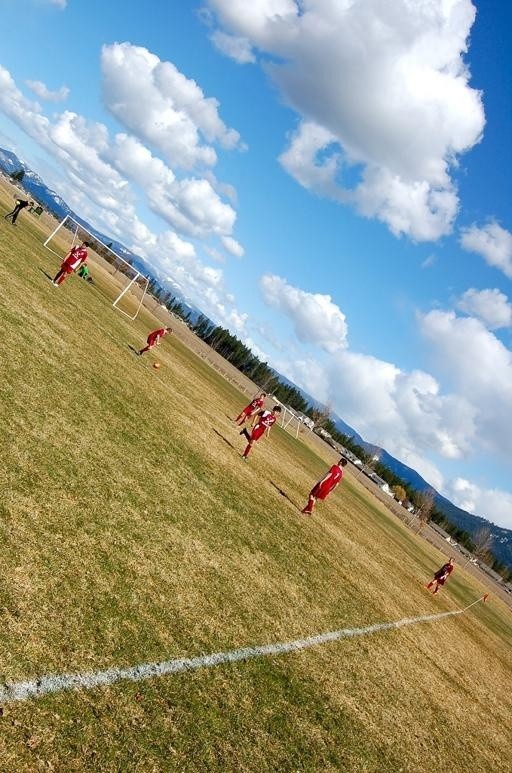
242, 455, 249, 463
433, 591, 437, 594
304, 509, 312, 514
240, 428, 246, 435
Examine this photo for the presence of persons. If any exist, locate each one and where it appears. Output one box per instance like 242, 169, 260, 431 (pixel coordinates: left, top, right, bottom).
240, 406, 281, 461
50, 241, 89, 287
4, 198, 35, 227
301, 458, 348, 515
74, 263, 88, 280
138, 327, 175, 358
424, 557, 455, 596
233, 393, 267, 427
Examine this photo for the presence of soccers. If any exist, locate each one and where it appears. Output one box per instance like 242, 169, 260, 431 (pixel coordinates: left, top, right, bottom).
154, 362, 160, 368
